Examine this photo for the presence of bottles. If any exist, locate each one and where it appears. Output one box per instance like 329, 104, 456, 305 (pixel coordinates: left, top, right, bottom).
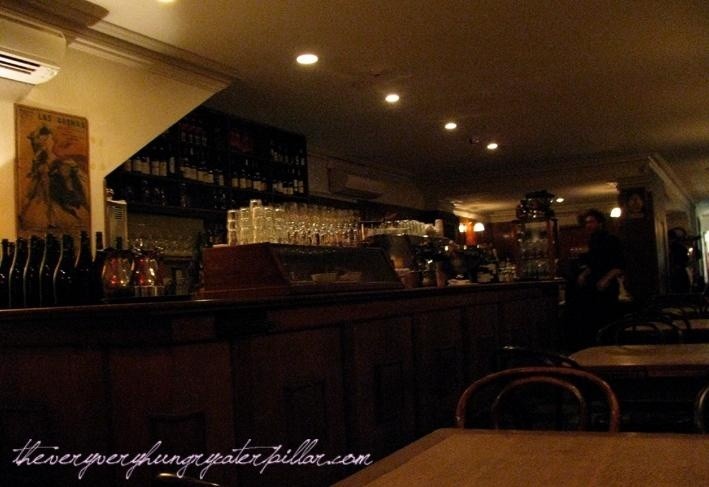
123, 123, 308, 214
0, 229, 166, 309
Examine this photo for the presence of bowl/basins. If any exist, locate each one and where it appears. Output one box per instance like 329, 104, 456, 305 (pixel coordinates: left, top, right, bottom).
310, 271, 337, 283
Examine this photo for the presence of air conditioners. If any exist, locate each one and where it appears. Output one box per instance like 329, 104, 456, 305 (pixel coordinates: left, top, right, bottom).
328, 164, 385, 199
0, 6, 66, 85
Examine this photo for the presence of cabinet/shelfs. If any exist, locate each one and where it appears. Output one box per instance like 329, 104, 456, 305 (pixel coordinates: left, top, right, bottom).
108, 106, 309, 219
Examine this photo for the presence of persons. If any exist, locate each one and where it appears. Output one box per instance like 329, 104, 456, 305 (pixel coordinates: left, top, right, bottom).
578, 208, 625, 346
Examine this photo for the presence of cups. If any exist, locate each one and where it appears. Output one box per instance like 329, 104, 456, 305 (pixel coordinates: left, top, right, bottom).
226, 198, 444, 251
